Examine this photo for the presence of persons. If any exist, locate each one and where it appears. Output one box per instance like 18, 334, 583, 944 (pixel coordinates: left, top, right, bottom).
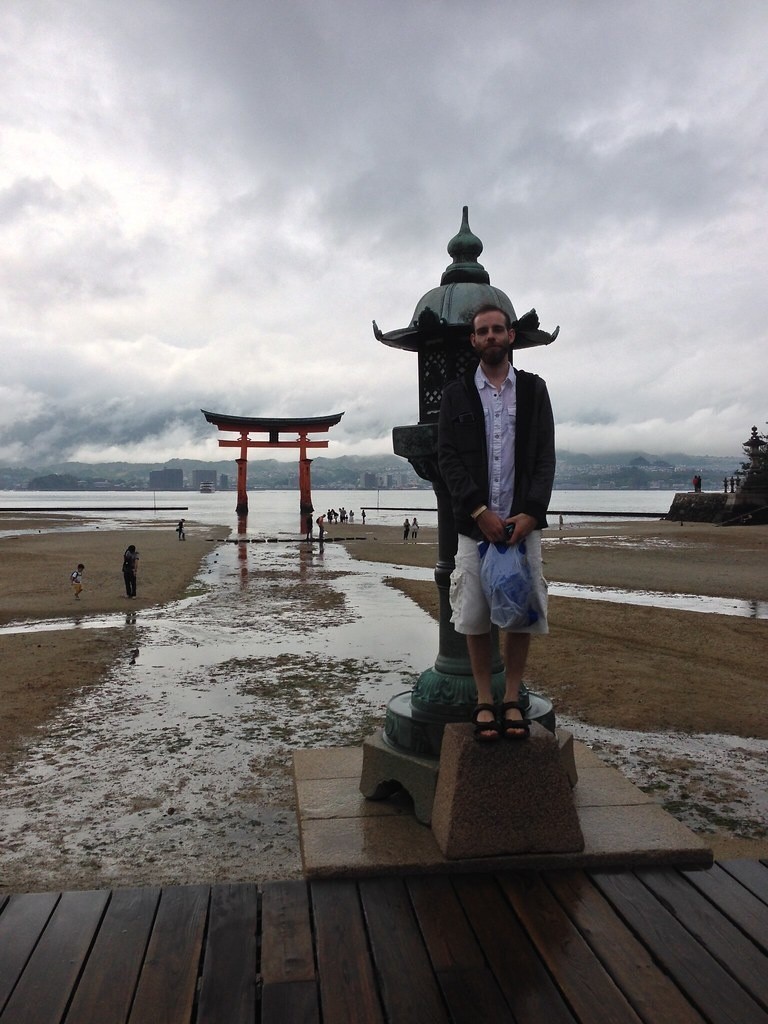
692, 475, 701, 492
123, 545, 139, 599
403, 518, 419, 544
306, 514, 314, 539
439, 304, 556, 746
327, 507, 354, 524
361, 510, 366, 524
558, 515, 563, 530
178, 519, 185, 541
71, 564, 85, 600
317, 514, 326, 539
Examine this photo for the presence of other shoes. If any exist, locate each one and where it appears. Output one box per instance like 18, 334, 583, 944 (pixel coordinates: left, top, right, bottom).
322, 537, 324, 538
125, 595, 129, 598
132, 596, 136, 600
76, 596, 80, 600
72, 593, 76, 596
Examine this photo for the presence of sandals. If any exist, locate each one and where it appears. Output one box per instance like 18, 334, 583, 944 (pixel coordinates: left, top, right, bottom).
500, 701, 532, 740
471, 702, 500, 741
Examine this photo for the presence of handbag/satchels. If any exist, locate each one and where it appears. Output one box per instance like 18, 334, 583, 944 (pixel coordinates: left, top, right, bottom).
479, 539, 541, 630
316, 517, 319, 523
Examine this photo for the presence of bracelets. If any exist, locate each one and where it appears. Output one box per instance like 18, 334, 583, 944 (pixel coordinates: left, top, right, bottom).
472, 505, 488, 519
